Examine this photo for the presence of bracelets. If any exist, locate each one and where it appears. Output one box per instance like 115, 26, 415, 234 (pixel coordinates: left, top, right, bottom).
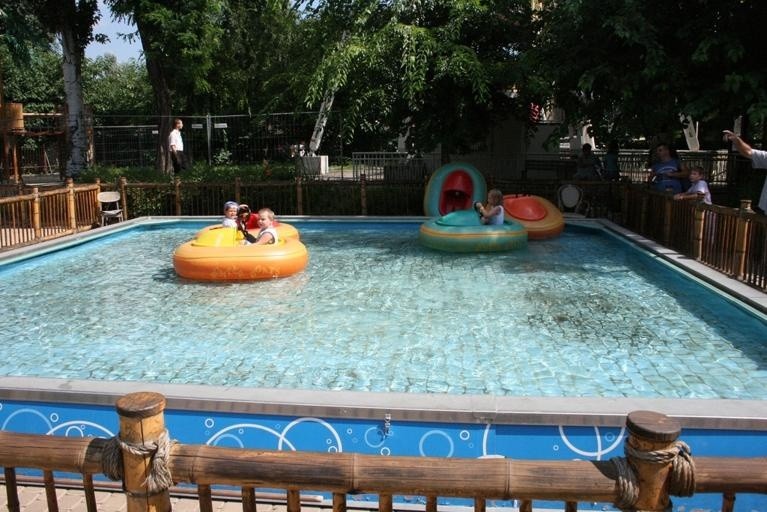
479, 207, 484, 212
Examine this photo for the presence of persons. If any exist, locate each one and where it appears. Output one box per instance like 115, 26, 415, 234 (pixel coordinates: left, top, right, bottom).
648, 143, 689, 196
167, 118, 192, 183
236, 208, 278, 248
473, 188, 505, 226
673, 166, 717, 259
604, 141, 621, 175
221, 200, 241, 230
573, 143, 602, 182
721, 127, 766, 215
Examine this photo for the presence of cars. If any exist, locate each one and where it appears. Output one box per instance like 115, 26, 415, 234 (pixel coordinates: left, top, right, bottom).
241, 135, 310, 164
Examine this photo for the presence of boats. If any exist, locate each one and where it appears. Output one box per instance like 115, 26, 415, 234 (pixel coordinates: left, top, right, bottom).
419, 208, 527, 252
192, 219, 300, 241
421, 161, 489, 223
167, 234, 310, 281
498, 192, 565, 241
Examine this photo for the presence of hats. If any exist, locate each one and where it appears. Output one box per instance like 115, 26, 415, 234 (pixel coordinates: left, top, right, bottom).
223, 201, 240, 211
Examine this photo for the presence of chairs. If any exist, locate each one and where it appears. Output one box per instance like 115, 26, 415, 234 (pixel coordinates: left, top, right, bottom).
96, 188, 128, 227
558, 180, 592, 224
